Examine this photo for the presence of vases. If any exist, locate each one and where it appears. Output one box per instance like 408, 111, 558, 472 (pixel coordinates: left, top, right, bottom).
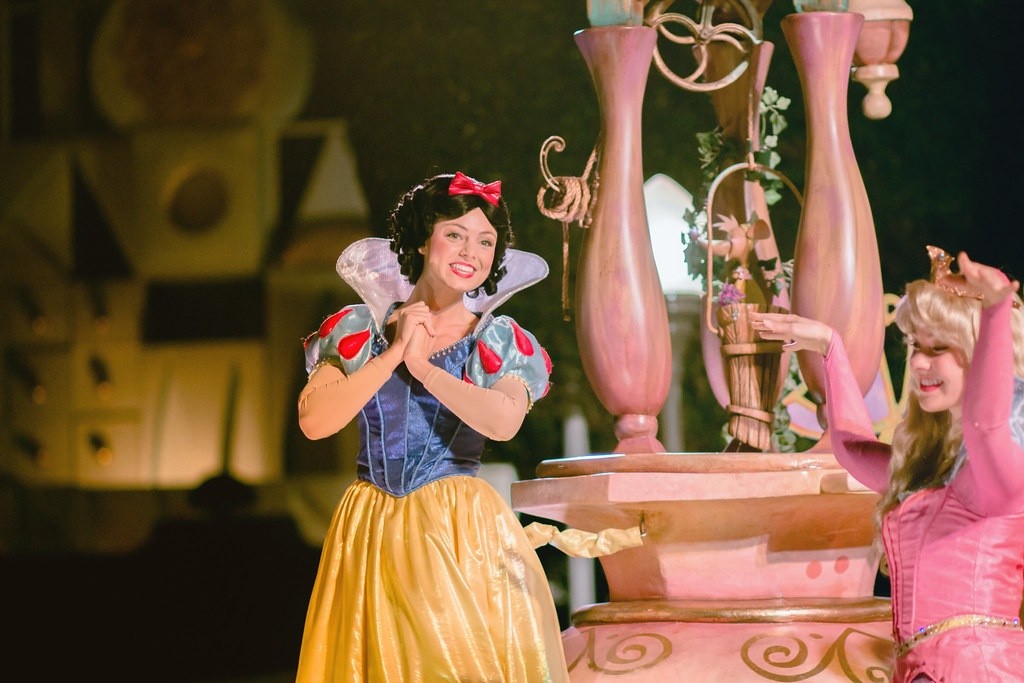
780, 12, 884, 427
571, 26, 672, 456
711, 303, 791, 453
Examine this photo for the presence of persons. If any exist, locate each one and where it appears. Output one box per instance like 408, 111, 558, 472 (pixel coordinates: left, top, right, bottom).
748, 246, 1024, 683
296, 171, 572, 683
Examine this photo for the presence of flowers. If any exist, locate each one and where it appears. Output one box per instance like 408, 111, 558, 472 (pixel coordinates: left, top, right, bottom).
688, 86, 796, 304
